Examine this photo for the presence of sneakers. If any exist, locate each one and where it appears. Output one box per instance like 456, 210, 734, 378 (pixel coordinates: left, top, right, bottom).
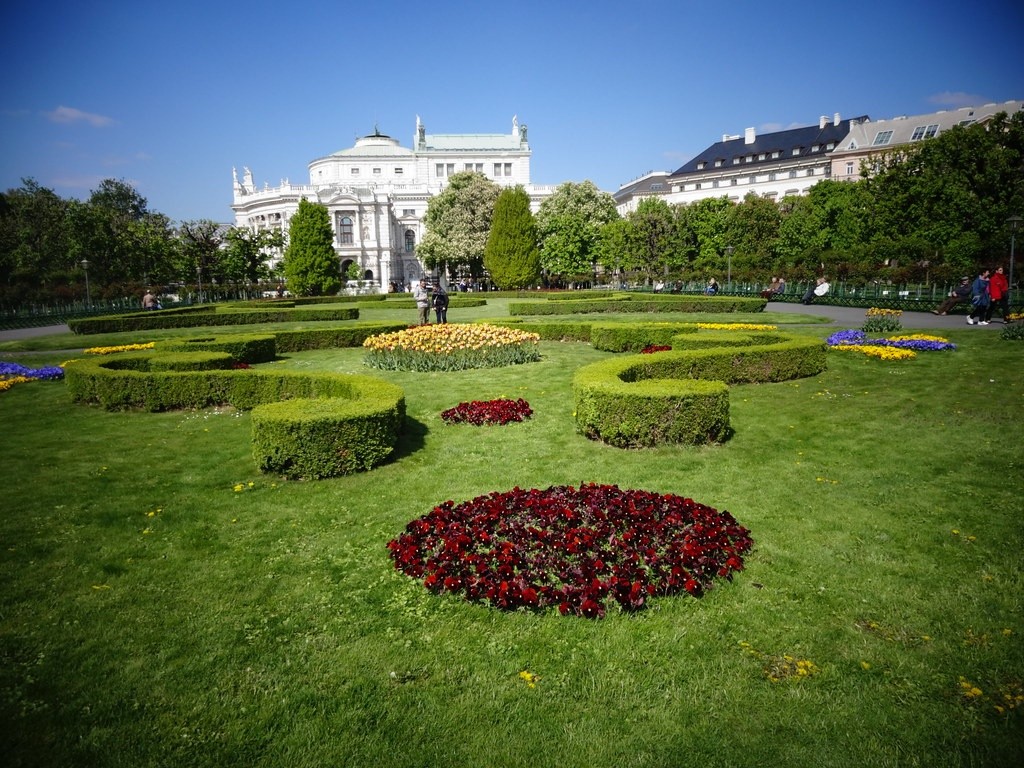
977, 320, 989, 326
966, 315, 974, 325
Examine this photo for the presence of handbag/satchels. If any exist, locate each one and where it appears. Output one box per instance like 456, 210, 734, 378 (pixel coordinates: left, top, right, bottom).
433, 300, 437, 306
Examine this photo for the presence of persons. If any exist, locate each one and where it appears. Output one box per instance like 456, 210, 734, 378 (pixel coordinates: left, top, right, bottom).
654, 279, 664, 292
760, 277, 785, 299
142, 290, 156, 311
431, 281, 449, 324
966, 266, 1009, 325
413, 279, 429, 325
799, 277, 829, 304
932, 276, 973, 316
708, 277, 718, 295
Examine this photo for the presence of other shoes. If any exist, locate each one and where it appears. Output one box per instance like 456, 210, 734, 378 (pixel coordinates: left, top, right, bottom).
941, 312, 947, 316
987, 321, 992, 323
798, 299, 808, 304
931, 310, 939, 315
1003, 319, 1014, 323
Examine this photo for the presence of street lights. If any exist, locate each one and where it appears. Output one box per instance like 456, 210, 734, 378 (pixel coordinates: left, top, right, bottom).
1004, 215, 1023, 304
81, 257, 93, 313
195, 266, 201, 302
726, 244, 734, 293
